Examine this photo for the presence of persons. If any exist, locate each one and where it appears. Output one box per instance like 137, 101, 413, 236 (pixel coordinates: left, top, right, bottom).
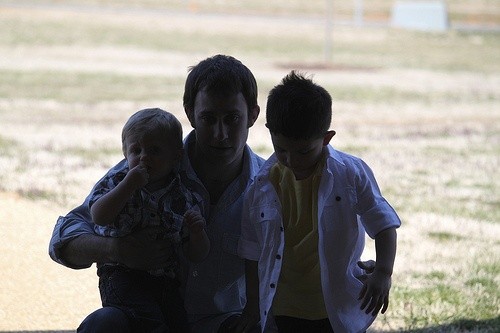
87, 107, 210, 332
49, 55, 377, 333
226, 69, 401, 333
